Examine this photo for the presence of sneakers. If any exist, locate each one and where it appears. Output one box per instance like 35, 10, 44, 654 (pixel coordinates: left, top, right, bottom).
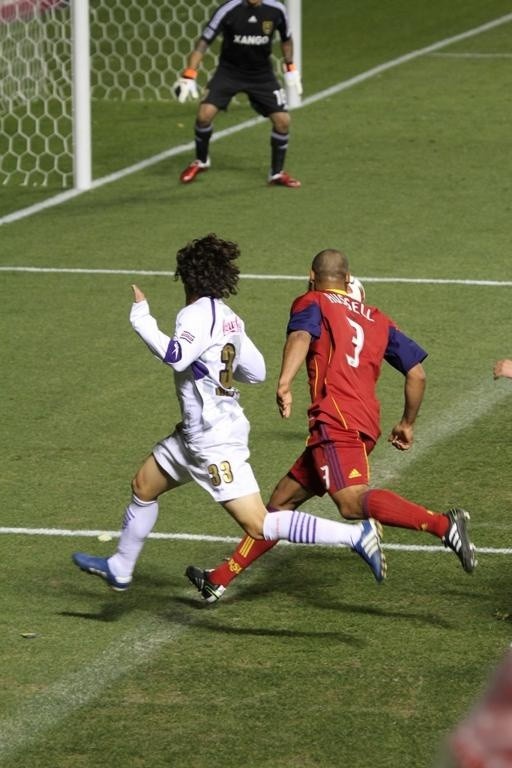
185, 566, 226, 604
441, 509, 479, 573
72, 553, 132, 593
269, 170, 302, 189
180, 154, 211, 183
350, 518, 388, 583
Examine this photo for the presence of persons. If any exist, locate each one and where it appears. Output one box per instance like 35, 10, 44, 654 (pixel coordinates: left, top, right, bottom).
170, 0, 302, 188
68, 232, 390, 593
492, 358, 512, 383
182, 246, 478, 603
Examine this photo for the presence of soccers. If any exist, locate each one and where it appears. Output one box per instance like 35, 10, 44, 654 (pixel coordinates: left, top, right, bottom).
346, 275, 365, 304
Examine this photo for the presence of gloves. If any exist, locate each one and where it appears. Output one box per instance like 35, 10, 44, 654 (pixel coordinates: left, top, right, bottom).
282, 61, 303, 96
172, 71, 198, 103
